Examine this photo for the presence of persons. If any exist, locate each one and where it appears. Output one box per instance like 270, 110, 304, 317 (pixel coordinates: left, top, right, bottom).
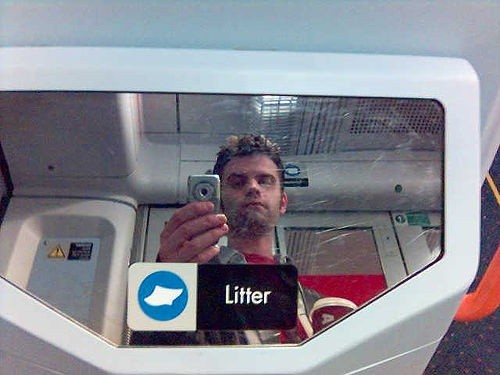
129, 134, 323, 347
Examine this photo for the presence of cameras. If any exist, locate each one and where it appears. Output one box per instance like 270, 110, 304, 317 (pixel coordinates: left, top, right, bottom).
188, 174, 221, 214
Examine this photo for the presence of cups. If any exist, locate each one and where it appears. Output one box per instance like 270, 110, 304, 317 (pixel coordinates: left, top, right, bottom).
309, 296, 358, 333
